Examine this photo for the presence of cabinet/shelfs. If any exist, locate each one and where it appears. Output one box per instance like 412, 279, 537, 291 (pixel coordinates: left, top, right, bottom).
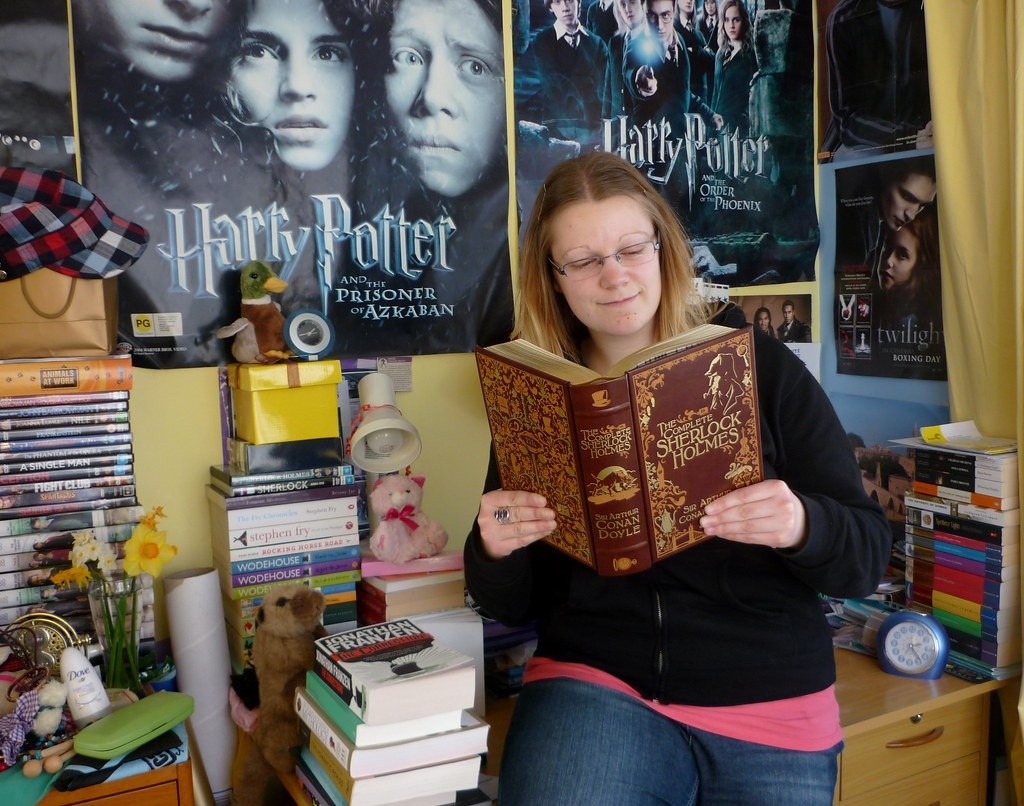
833, 645, 1010, 806
38, 684, 194, 806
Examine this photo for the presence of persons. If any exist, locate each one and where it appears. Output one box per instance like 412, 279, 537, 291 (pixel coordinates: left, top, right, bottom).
73, 0, 276, 365
532, 0, 758, 211
818, 0, 935, 161
776, 300, 806, 343
873, 205, 946, 379
331, 0, 513, 356
836, 158, 936, 291
455, 151, 892, 805
226, 0, 371, 287
752, 307, 774, 336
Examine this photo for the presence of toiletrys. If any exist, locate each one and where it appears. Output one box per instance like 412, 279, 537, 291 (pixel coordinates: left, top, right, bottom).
59, 645, 114, 731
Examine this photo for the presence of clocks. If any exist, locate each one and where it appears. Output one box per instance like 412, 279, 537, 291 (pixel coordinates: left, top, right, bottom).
878, 607, 951, 680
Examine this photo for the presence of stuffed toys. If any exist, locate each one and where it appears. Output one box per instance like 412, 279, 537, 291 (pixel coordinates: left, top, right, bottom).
368, 474, 448, 562
215, 259, 294, 365
231, 584, 329, 806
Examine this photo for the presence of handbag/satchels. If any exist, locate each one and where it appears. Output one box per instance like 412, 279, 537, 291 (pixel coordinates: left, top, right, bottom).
1, 268, 119, 357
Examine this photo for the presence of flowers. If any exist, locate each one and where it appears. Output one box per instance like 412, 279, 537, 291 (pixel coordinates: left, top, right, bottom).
50, 504, 179, 692
87, 581, 144, 696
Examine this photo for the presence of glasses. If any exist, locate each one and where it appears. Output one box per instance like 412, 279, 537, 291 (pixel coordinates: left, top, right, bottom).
646, 12, 673, 24
546, 228, 661, 282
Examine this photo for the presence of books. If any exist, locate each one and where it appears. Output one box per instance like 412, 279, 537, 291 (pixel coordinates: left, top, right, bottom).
474, 325, 764, 578
289, 619, 489, 806
819, 419, 1021, 684
0, 354, 156, 664
205, 466, 465, 676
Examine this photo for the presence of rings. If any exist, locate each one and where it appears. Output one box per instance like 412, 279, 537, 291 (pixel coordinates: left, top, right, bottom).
495, 506, 511, 524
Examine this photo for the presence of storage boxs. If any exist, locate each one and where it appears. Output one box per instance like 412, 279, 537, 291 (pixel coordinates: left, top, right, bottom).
226, 436, 343, 474
227, 359, 344, 446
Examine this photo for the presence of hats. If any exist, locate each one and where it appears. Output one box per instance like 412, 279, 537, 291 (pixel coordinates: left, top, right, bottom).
0, 164, 150, 283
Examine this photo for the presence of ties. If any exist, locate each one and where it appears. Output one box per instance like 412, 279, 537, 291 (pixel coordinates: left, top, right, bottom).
622, 31, 631, 83
602, 2, 605, 13
686, 22, 693, 34
667, 36, 677, 66
721, 45, 734, 64
565, 31, 580, 49
708, 18, 714, 38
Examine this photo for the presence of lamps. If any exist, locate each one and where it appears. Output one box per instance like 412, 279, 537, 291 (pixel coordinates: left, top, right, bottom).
350, 370, 423, 537
